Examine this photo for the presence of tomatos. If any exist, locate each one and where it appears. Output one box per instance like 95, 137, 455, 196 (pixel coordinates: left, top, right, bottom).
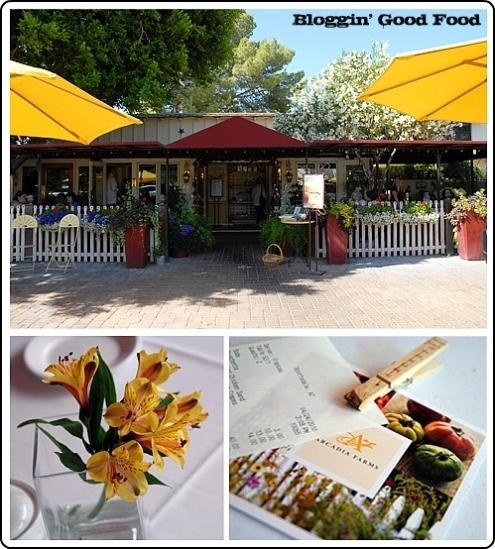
382, 412, 425, 445
407, 398, 451, 427
415, 443, 463, 483
424, 421, 477, 461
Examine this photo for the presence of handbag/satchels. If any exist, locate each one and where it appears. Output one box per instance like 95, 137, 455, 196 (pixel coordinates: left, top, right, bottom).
259, 193, 266, 207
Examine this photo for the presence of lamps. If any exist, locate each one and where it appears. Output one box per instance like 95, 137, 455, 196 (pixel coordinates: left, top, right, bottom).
183, 169, 191, 184
286, 168, 293, 182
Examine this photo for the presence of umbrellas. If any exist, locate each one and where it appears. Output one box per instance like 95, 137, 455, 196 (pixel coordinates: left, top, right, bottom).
126, 166, 160, 187
9, 58, 142, 147
358, 39, 488, 123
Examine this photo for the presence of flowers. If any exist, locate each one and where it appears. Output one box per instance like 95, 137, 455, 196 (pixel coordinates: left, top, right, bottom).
170, 224, 196, 252
404, 200, 435, 214
36, 208, 75, 223
84, 210, 112, 225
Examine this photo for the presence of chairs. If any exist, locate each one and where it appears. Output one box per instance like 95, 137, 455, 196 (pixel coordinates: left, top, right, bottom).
10, 214, 38, 274
45, 214, 80, 274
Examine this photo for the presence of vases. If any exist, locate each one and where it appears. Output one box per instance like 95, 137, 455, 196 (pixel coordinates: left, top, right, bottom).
397, 211, 438, 224
84, 222, 109, 233
39, 222, 67, 232
173, 246, 188, 257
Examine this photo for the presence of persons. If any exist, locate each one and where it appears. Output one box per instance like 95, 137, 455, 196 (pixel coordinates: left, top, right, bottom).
403, 191, 412, 202
105, 169, 120, 207
251, 180, 267, 226
11, 191, 26, 205
25, 194, 35, 203
65, 191, 77, 204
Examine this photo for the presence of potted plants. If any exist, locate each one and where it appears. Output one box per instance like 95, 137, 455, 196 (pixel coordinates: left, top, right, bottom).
326, 202, 355, 264
260, 219, 308, 257
153, 247, 166, 265
447, 187, 487, 261
359, 204, 393, 226
109, 198, 154, 268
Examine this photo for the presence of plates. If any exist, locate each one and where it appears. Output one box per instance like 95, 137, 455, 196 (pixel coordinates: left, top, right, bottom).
22, 336, 143, 385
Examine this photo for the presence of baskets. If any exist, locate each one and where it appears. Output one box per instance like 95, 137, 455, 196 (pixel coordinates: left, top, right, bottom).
262, 243, 285, 268
